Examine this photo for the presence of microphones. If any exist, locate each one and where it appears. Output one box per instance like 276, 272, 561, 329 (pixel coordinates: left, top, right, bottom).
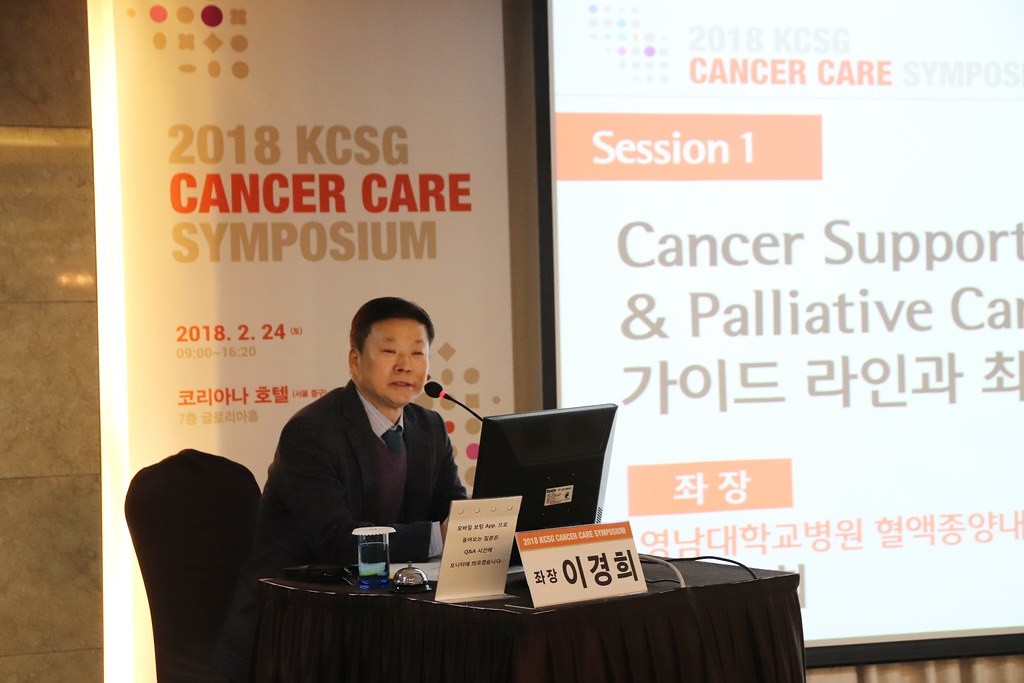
424, 382, 483, 423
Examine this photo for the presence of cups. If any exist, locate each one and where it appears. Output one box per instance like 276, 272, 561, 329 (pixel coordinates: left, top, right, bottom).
358, 531, 390, 591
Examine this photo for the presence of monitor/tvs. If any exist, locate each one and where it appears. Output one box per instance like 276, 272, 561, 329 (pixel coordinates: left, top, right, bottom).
471, 403, 618, 566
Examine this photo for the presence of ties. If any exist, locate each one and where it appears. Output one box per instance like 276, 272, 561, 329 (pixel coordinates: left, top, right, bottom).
381, 425, 402, 456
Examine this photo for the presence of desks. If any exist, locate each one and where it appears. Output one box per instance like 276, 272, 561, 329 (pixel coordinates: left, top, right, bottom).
252, 557, 806, 683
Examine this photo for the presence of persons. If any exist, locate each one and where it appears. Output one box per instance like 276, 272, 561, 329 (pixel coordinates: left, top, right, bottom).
207, 298, 467, 682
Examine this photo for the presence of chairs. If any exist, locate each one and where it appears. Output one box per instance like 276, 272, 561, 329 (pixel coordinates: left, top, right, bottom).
124, 449, 264, 683
